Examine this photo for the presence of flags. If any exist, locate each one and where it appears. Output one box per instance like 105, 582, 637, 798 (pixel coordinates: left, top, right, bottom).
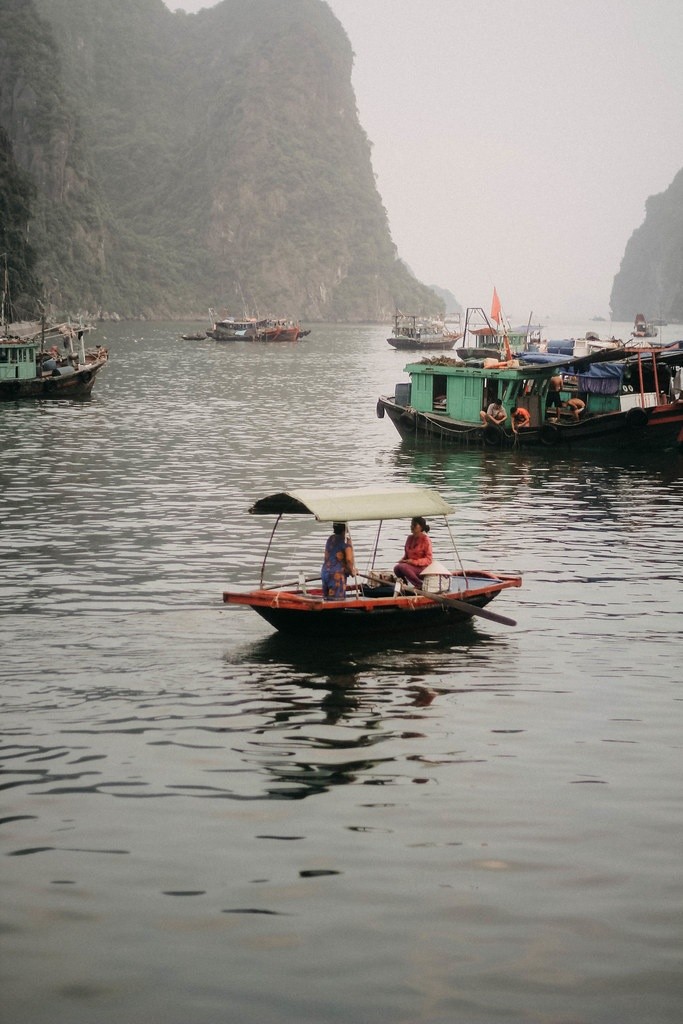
491, 286, 502, 324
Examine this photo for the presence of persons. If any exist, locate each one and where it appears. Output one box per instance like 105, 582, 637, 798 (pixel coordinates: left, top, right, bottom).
545, 373, 563, 422
321, 520, 359, 600
509, 407, 531, 434
480, 398, 507, 428
560, 397, 585, 422
393, 517, 432, 590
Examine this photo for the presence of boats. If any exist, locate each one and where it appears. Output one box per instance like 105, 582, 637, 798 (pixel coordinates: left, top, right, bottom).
0, 253, 109, 395
223, 632, 500, 666
181, 297, 312, 341
223, 488, 522, 644
377, 285, 683, 441
